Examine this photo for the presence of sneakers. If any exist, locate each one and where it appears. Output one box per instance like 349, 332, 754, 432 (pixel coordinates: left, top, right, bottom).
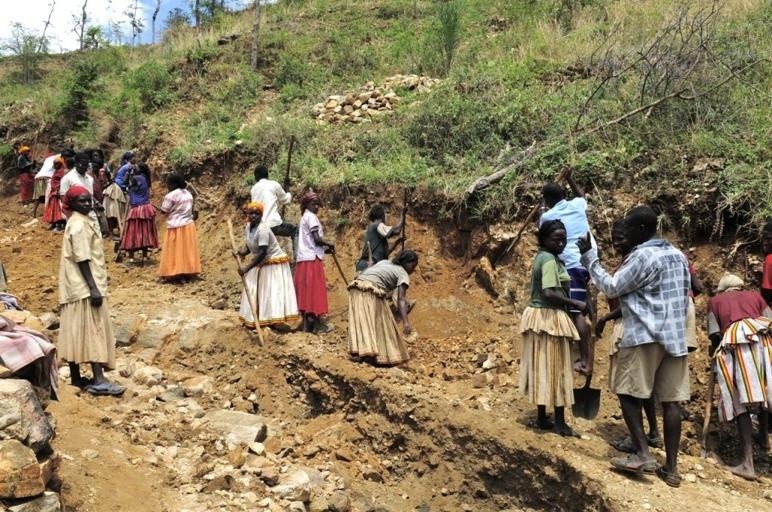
610, 438, 637, 453
646, 436, 660, 448
87, 383, 127, 394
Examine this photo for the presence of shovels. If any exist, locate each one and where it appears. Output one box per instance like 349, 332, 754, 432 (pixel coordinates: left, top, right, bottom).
571, 283, 601, 420
114, 196, 130, 253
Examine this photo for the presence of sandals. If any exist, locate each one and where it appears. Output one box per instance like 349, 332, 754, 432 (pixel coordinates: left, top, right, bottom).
610, 454, 656, 474
656, 467, 681, 488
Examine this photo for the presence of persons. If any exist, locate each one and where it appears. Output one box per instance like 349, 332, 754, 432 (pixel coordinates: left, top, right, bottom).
249, 165, 299, 262
518, 219, 591, 438
706, 270, 772, 481
155, 174, 200, 284
575, 204, 702, 488
16, 146, 136, 239
345, 250, 419, 366
293, 191, 337, 335
356, 206, 407, 269
538, 166, 599, 376
115, 163, 159, 266
57, 185, 128, 398
232, 203, 300, 330
